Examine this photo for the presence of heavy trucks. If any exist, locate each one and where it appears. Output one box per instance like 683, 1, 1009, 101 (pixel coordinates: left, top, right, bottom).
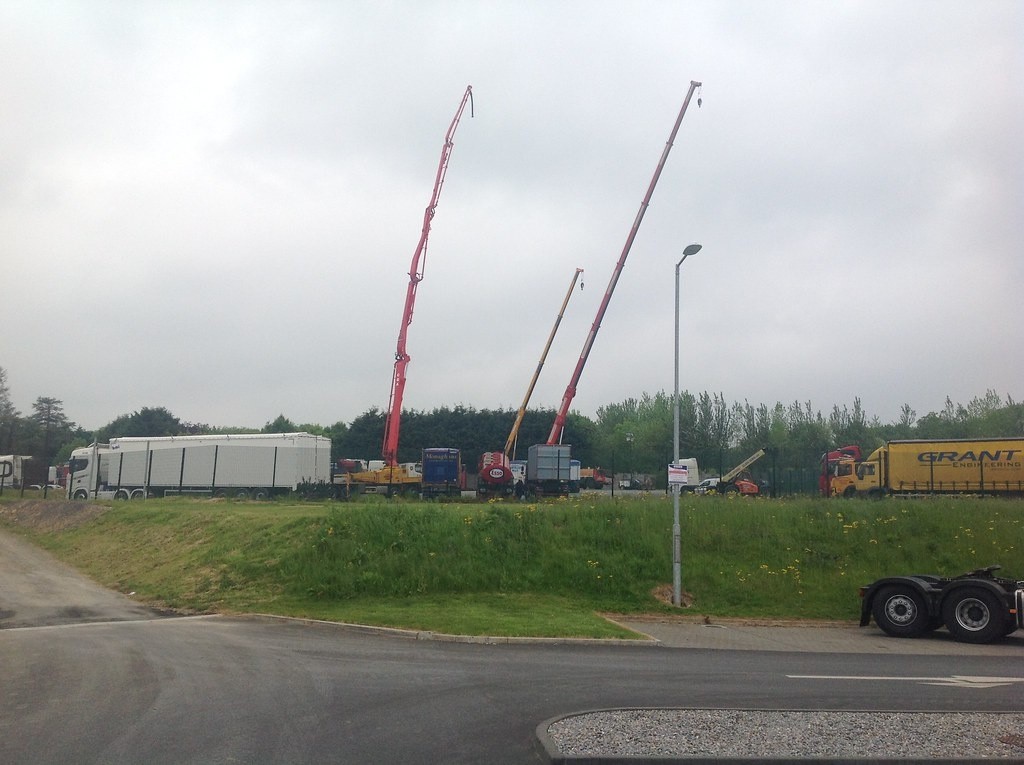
817, 437, 1024, 501
65, 428, 334, 502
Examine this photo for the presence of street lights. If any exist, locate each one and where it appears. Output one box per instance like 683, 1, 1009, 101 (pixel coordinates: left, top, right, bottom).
668, 244, 703, 607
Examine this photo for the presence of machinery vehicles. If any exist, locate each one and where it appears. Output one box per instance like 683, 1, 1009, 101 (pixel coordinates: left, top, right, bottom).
343, 80, 777, 501
